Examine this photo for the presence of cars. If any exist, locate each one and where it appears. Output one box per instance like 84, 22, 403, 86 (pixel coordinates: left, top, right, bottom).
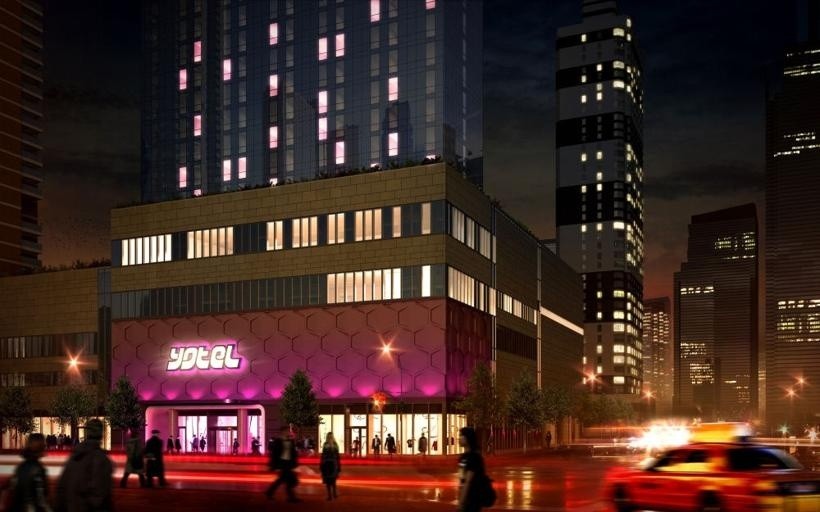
603, 422, 820, 511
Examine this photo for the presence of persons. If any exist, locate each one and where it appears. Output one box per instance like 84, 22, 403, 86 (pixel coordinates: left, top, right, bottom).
320, 432, 341, 500
457, 427, 486, 512
232, 438, 237, 456
1, 256, 110, 276
353, 433, 396, 459
295, 435, 316, 455
545, 430, 552, 449
10, 433, 54, 512
267, 427, 304, 504
45, 433, 78, 451
111, 151, 539, 240
53, 419, 118, 512
416, 432, 427, 456
251, 435, 262, 455
167, 435, 182, 453
120, 428, 169, 489
190, 435, 206, 455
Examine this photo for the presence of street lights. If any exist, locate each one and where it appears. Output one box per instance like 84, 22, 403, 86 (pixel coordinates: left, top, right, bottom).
378, 341, 404, 458
64, 356, 108, 452
